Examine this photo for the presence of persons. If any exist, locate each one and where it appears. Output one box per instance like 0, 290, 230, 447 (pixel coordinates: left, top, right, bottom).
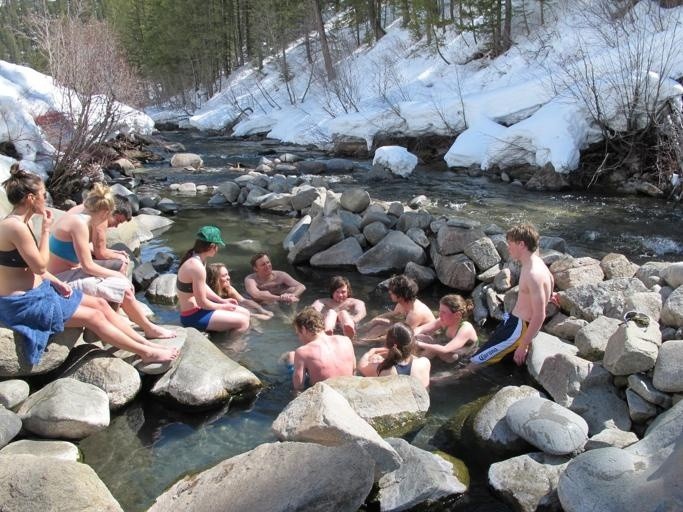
0, 164, 181, 366
292, 308, 357, 390
430, 224, 554, 384
175, 225, 306, 335
46, 179, 177, 339
310, 276, 366, 338
67, 195, 128, 311
357, 276, 478, 388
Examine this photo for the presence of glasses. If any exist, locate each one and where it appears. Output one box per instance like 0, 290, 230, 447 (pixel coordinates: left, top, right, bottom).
36, 191, 48, 199
624, 311, 650, 324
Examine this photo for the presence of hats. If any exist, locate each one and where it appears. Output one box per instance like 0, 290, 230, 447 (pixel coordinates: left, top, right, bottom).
197, 225, 226, 247
